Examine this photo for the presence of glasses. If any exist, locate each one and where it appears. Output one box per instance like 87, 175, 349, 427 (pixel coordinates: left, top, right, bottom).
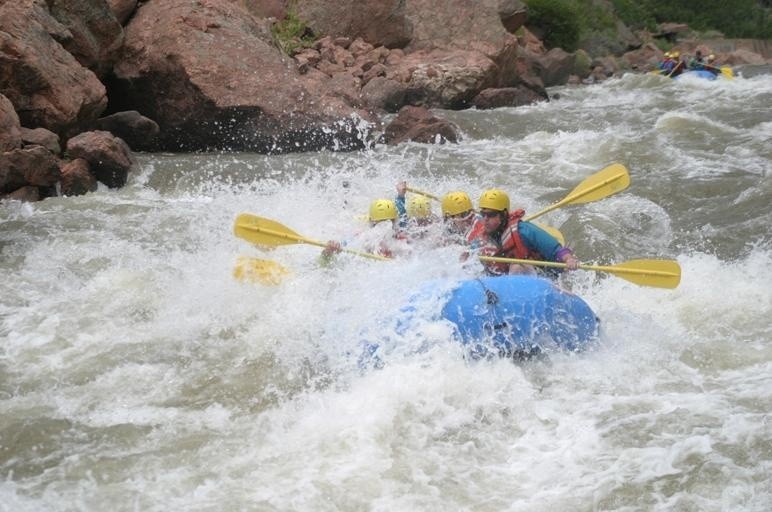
479, 209, 501, 219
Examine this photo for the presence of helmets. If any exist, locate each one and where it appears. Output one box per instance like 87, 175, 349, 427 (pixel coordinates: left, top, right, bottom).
406, 196, 433, 219
663, 51, 681, 59
441, 191, 473, 217
368, 199, 398, 222
477, 188, 511, 214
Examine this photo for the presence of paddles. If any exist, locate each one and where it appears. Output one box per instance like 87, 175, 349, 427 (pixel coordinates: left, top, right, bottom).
650, 69, 666, 75
232, 256, 293, 288
475, 258, 682, 289
233, 213, 393, 263
517, 162, 631, 223
404, 182, 566, 249
696, 62, 735, 81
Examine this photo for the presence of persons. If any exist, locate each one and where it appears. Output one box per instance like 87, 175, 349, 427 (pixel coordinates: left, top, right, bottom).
656, 50, 721, 77
318, 180, 582, 282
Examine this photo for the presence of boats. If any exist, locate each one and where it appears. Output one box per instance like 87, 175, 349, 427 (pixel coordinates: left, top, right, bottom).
671, 70, 717, 81
356, 276, 601, 383
